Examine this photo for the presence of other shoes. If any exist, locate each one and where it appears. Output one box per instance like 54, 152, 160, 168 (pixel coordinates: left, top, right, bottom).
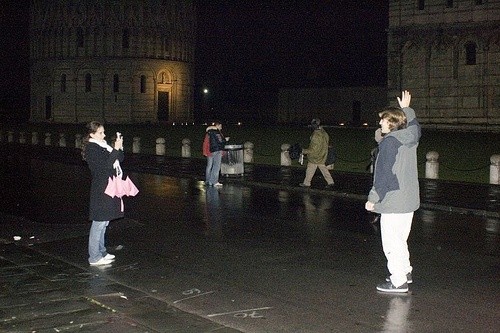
104, 254, 116, 259
89, 257, 114, 265
216, 182, 223, 185
299, 183, 310, 187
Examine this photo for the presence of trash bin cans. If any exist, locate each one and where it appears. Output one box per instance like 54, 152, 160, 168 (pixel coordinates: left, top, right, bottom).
221, 145, 244, 177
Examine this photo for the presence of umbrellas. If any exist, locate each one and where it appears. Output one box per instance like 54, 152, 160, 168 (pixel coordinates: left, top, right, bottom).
104, 166, 139, 212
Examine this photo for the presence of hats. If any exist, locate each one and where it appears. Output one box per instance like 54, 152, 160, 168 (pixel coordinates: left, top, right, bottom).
311, 118, 320, 125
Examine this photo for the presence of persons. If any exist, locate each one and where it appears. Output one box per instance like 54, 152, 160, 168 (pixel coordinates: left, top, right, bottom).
299, 117, 336, 188
202, 121, 226, 186
80, 121, 126, 266
365, 90, 421, 292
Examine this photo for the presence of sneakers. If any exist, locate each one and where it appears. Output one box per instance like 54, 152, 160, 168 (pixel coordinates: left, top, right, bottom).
376, 282, 408, 293
386, 273, 413, 283
